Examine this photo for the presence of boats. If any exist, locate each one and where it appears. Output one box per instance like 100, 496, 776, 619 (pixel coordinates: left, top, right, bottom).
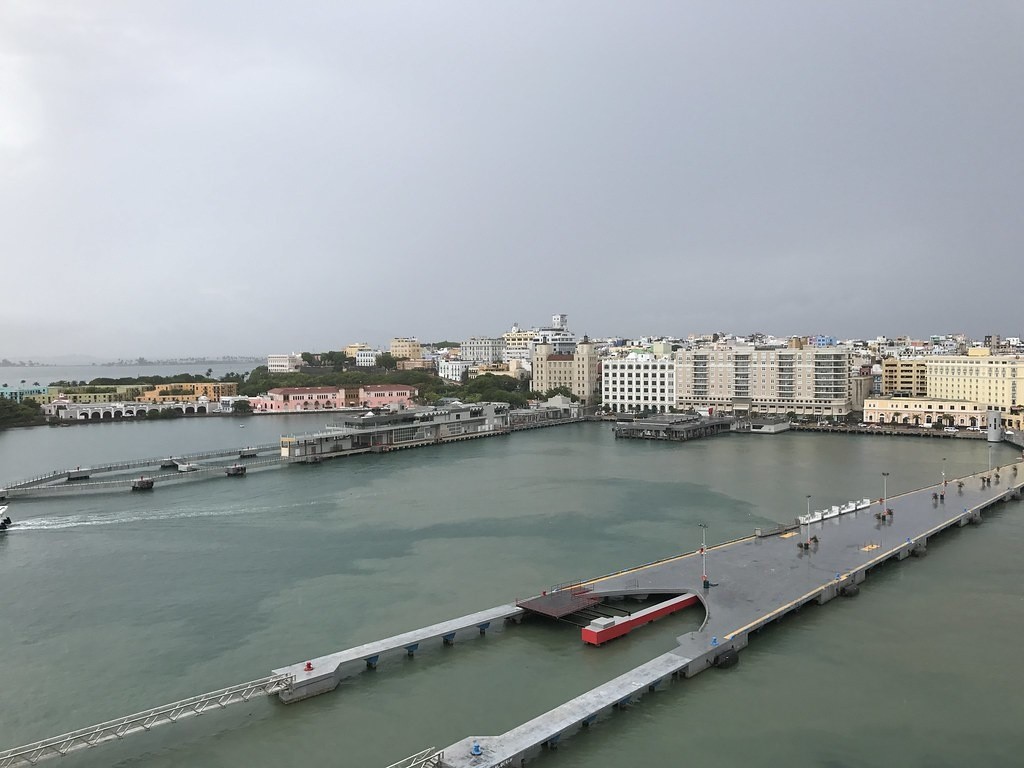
240, 423, 245, 428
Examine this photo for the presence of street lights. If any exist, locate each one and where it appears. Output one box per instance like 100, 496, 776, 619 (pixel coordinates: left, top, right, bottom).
805, 494, 812, 545
881, 472, 890, 520
698, 522, 708, 582
942, 456, 947, 496
988, 445, 993, 480
